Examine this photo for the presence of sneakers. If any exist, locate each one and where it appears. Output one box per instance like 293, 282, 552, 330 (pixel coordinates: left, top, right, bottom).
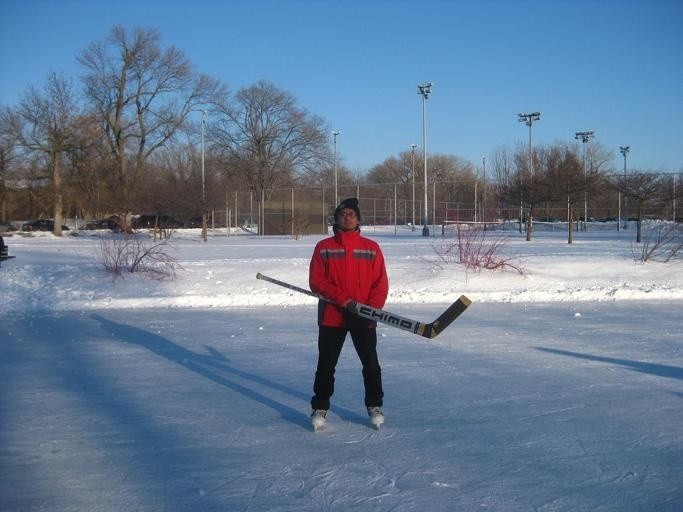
367, 406, 385, 424
310, 409, 327, 426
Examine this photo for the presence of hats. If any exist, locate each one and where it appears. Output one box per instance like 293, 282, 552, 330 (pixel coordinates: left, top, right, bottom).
335, 198, 360, 214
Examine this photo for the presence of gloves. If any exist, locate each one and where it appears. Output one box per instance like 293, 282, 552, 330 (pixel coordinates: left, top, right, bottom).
345, 300, 358, 314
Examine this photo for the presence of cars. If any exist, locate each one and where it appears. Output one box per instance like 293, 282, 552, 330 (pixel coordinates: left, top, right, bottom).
87, 220, 122, 230
22, 219, 70, 231
132, 214, 224, 228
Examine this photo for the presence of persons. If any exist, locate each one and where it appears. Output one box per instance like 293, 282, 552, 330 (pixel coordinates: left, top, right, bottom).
309, 198, 388, 425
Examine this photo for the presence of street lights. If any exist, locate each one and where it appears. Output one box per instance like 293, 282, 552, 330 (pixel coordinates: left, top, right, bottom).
575, 131, 630, 230
482, 155, 487, 206
517, 112, 541, 232
332, 127, 341, 209
201, 110, 208, 228
410, 82, 433, 225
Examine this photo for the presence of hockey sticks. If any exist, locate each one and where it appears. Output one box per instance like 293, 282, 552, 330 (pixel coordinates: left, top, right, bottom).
256, 273, 471, 339
